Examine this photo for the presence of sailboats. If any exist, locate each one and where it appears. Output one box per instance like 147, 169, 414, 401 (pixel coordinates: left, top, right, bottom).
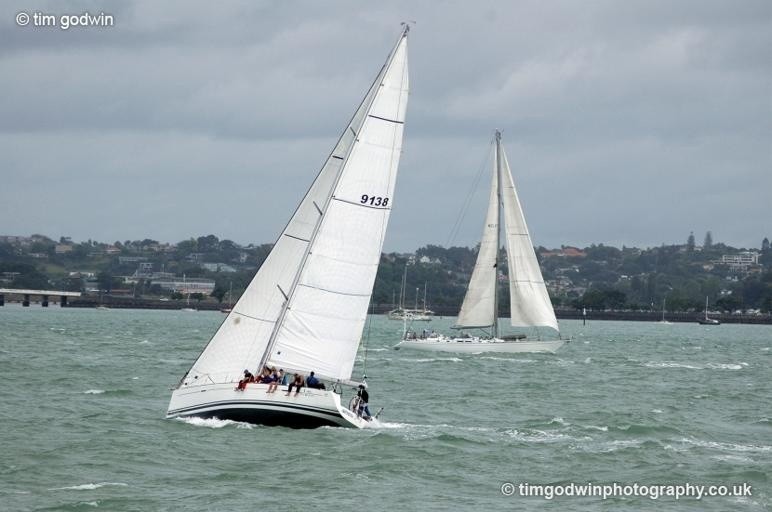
393, 128, 573, 354
165, 22, 413, 427
387, 265, 435, 321
696, 295, 721, 324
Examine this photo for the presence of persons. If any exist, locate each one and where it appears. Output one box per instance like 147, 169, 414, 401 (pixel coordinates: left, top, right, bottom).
405, 330, 438, 340
234, 368, 255, 393
357, 385, 372, 417
255, 365, 326, 397
